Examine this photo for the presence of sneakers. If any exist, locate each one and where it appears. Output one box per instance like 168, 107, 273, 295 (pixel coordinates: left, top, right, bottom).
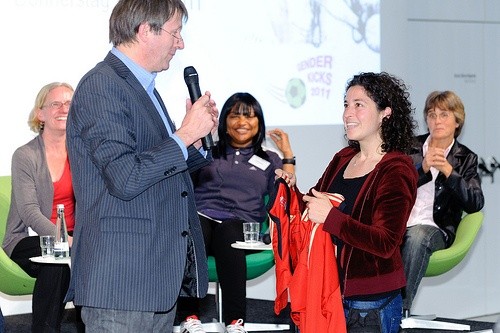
179, 318, 206, 333
224, 319, 248, 333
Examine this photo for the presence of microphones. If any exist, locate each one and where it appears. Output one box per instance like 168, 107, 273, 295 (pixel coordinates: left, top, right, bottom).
184, 65, 215, 150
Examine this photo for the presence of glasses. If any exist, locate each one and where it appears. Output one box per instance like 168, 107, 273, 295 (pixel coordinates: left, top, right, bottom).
154, 25, 182, 45
41, 101, 69, 109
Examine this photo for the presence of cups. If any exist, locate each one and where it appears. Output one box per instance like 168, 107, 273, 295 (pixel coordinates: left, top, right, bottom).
39, 236, 54, 257
243, 223, 259, 242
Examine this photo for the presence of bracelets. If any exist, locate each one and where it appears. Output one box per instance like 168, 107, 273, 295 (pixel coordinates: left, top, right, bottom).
281, 157, 296, 166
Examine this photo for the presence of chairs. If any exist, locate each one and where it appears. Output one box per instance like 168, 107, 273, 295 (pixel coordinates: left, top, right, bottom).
399, 208, 485, 331
1, 175, 36, 295
171, 192, 291, 333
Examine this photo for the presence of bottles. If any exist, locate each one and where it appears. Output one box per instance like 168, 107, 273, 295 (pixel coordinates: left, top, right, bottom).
53, 204, 69, 260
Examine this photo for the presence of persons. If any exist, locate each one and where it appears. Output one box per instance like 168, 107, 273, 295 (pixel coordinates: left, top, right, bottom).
274, 72, 420, 333
2, 83, 85, 333
178, 92, 296, 333
402, 89, 485, 318
62, 0, 219, 333
